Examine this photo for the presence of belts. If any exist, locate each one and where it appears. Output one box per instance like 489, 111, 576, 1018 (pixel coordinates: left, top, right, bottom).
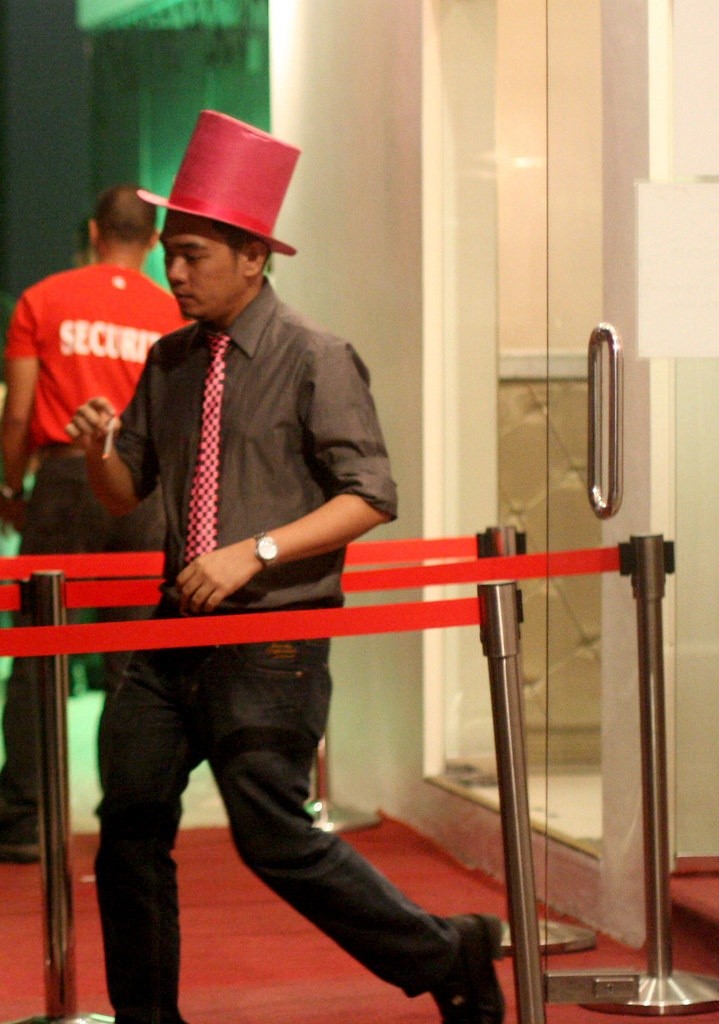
40, 444, 85, 459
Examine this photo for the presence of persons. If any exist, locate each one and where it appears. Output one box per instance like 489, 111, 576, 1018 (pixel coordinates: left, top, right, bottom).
0, 181, 201, 864
64, 179, 511, 1024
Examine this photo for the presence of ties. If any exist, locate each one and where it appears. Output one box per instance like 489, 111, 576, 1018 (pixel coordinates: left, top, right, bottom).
186, 327, 232, 564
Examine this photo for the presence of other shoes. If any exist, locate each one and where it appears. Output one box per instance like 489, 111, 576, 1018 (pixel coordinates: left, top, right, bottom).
1, 835, 42, 863
432, 910, 506, 1024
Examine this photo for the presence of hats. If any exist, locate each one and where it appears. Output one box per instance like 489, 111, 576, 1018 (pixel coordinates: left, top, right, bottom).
133, 110, 302, 257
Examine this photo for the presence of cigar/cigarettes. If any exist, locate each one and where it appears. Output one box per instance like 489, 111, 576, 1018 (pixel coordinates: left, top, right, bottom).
102, 418, 116, 461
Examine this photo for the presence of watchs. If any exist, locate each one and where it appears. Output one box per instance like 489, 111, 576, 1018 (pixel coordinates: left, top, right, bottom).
253, 531, 279, 569
1, 484, 24, 500
